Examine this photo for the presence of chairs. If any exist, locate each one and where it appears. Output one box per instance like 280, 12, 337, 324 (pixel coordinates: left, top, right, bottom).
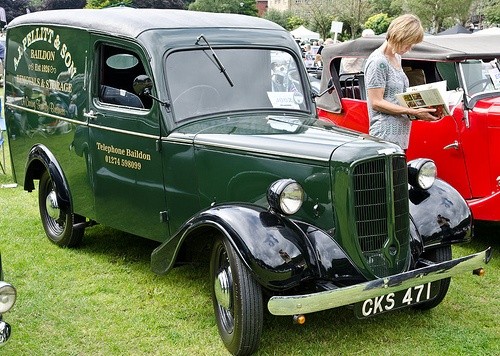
404, 60, 462, 92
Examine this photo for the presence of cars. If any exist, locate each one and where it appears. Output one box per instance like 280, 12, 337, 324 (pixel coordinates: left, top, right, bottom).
270, 28, 500, 222
5, 8, 491, 356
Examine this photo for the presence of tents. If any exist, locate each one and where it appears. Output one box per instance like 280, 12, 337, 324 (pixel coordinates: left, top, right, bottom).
290, 25, 320, 45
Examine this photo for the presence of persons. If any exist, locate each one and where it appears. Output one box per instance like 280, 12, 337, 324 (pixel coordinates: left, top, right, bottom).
364, 14, 440, 156
303, 38, 340, 67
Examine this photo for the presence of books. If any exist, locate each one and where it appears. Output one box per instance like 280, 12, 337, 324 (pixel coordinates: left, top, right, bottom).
395, 80, 464, 122
